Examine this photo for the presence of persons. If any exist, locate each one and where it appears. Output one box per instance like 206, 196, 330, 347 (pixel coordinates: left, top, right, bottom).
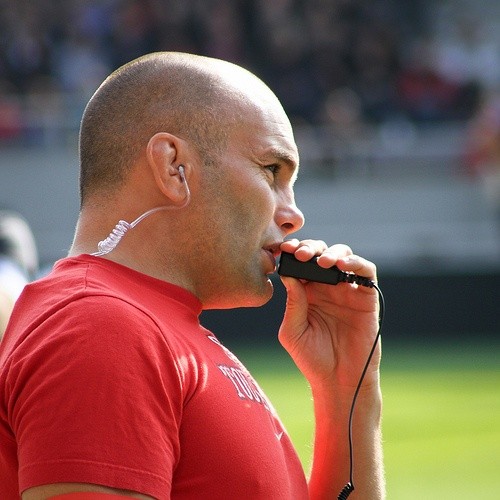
0, 51, 389, 500
0, 214, 39, 341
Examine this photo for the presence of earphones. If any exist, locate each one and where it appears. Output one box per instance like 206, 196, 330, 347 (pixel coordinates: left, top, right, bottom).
178, 165, 185, 179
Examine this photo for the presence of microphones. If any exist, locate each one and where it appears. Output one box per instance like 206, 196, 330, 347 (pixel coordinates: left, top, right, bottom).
277, 252, 341, 285
